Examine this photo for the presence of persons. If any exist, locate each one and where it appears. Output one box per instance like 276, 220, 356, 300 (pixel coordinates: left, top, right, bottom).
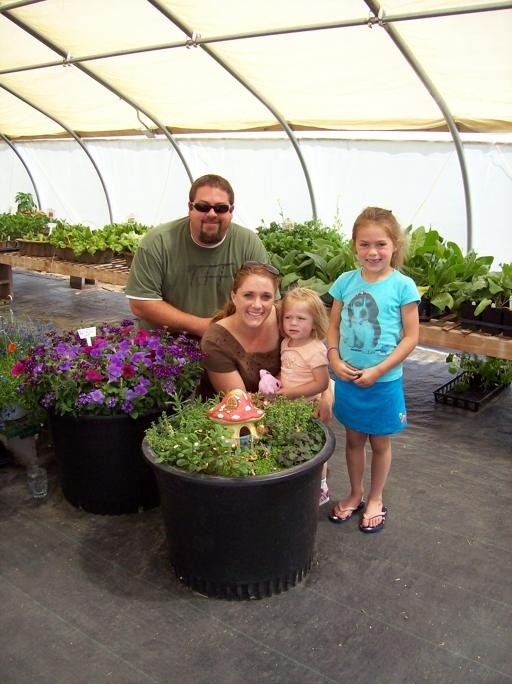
201, 257, 285, 400
273, 286, 336, 508
327, 207, 421, 534
125, 175, 271, 404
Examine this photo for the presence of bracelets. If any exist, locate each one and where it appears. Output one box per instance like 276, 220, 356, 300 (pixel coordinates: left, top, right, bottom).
326, 346, 339, 362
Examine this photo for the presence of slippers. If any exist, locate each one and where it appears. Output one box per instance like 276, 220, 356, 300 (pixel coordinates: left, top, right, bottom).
359, 507, 387, 532
329, 499, 365, 523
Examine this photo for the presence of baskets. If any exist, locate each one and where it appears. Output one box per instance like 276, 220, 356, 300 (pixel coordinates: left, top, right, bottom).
433, 370, 507, 412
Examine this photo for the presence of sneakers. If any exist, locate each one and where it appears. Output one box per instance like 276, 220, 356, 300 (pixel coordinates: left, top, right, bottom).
319, 488, 330, 506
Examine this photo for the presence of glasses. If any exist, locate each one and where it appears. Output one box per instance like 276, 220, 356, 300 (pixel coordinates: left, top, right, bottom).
191, 202, 230, 213
241, 260, 280, 277
